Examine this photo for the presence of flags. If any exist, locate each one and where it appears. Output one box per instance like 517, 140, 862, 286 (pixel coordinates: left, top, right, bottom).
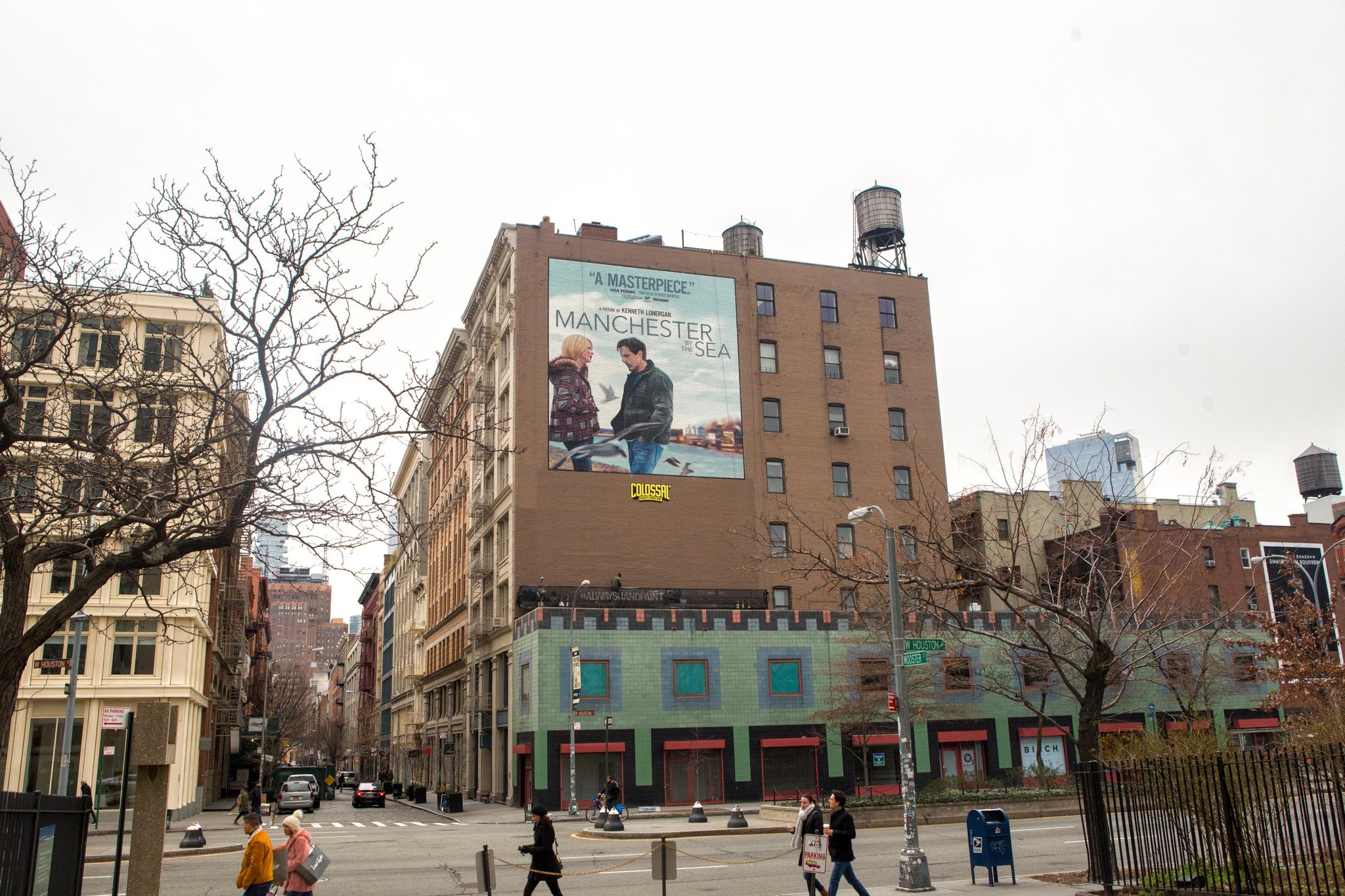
571, 646, 581, 704
304, 747, 327, 765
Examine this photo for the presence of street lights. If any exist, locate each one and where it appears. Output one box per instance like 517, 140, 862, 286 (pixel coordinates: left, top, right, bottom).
849, 507, 936, 892
1249, 539, 1344, 761
568, 579, 590, 818
258, 646, 385, 808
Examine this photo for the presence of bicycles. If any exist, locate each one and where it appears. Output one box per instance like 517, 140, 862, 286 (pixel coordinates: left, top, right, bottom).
586, 794, 628, 823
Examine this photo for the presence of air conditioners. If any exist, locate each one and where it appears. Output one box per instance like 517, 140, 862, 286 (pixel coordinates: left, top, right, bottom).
492, 619, 505, 625
1205, 559, 1216, 567
1248, 604, 1259, 611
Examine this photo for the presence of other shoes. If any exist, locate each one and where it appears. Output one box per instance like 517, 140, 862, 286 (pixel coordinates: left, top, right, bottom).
260, 823, 268, 826
340, 791, 342, 793
89, 821, 96, 825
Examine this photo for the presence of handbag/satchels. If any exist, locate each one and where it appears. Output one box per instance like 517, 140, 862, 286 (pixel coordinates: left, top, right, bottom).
271, 845, 288, 884
555, 854, 564, 870
295, 842, 330, 887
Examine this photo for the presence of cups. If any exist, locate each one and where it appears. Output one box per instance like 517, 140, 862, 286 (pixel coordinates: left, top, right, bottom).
823, 824, 830, 829
787, 823, 791, 828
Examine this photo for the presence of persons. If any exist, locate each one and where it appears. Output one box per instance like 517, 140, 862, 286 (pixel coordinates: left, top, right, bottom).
441, 782, 446, 792
80, 779, 97, 826
598, 775, 621, 811
336, 766, 349, 773
249, 781, 268, 827
518, 805, 564, 896
233, 785, 249, 825
273, 809, 316, 896
823, 789, 870, 896
378, 768, 394, 788
236, 812, 274, 896
338, 773, 345, 793
786, 793, 829, 896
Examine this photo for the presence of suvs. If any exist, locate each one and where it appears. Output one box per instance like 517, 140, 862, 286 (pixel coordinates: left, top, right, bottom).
278, 780, 314, 813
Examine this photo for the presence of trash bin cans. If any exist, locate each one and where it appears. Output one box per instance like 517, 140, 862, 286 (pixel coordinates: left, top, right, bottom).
966, 808, 1017, 887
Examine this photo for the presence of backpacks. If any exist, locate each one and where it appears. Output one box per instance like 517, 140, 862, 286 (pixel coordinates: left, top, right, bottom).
248, 787, 256, 801
236, 792, 245, 805
617, 783, 621, 791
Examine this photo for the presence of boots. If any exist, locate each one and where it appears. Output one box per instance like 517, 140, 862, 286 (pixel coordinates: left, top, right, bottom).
819, 886, 828, 896
233, 820, 239, 825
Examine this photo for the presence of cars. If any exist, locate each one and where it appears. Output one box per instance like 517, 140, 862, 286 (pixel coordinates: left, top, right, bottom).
97, 773, 137, 796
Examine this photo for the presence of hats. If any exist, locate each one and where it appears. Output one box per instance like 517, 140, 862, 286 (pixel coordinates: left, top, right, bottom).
531, 804, 548, 816
282, 809, 303, 831
